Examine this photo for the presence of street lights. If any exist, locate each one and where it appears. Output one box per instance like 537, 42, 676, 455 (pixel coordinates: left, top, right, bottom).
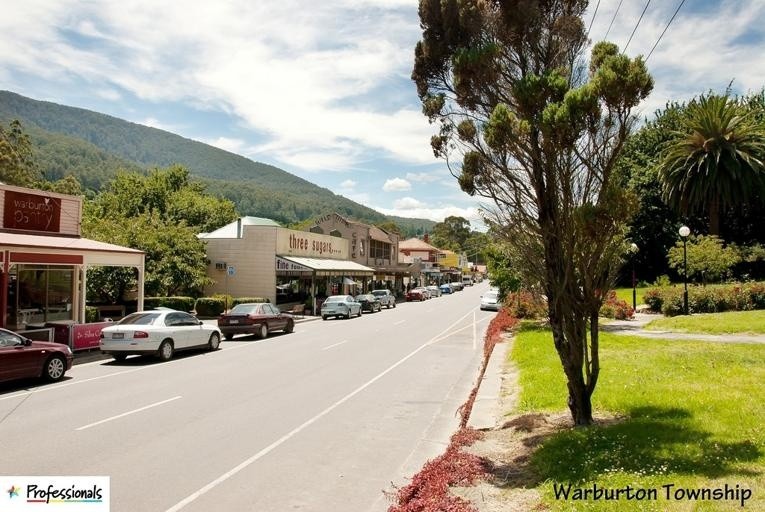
629, 241, 640, 312
679, 224, 692, 314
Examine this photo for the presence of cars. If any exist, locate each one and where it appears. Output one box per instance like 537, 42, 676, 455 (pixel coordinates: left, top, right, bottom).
0, 326, 74, 388
97, 309, 222, 362
479, 291, 500, 312
404, 274, 475, 301
216, 302, 295, 340
322, 287, 397, 319
